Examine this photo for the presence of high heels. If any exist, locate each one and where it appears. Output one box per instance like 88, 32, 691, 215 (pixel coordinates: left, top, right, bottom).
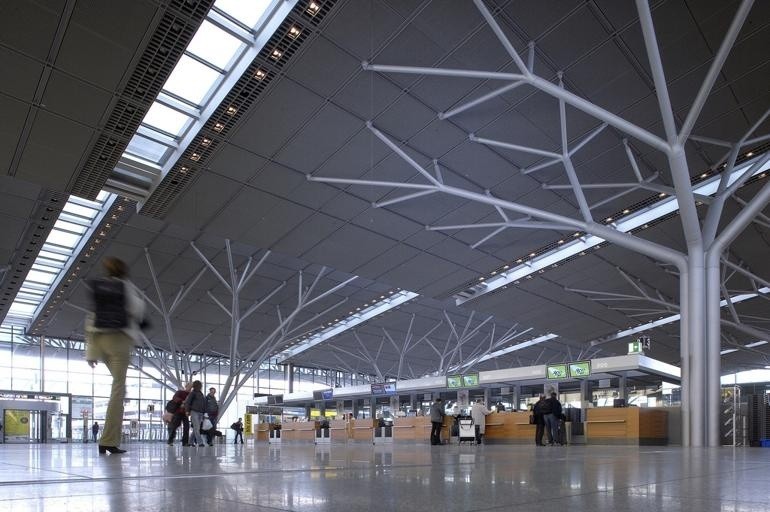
97, 444, 128, 455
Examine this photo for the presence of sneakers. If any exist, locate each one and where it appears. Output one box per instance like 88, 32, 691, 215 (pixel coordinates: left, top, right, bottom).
168, 439, 244, 448
536, 441, 563, 447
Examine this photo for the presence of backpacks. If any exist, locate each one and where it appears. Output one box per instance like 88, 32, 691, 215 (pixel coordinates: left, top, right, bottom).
431, 434, 437, 445
541, 397, 554, 415
92, 277, 130, 330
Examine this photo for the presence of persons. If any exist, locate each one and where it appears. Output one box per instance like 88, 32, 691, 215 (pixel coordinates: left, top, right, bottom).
185, 380, 206, 447
160, 445, 244, 511
234, 418, 244, 443
532, 395, 546, 447
166, 382, 193, 446
348, 412, 354, 419
429, 399, 444, 445
472, 399, 494, 445
206, 388, 218, 446
92, 421, 100, 442
84, 257, 149, 454
543, 392, 567, 446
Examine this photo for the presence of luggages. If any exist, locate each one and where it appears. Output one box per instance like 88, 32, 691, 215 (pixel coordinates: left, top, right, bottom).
558, 414, 568, 446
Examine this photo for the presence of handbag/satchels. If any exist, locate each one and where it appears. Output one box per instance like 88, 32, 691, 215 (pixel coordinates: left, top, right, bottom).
231, 422, 239, 430
201, 411, 214, 431
162, 412, 176, 422
166, 400, 181, 413
529, 413, 537, 424
